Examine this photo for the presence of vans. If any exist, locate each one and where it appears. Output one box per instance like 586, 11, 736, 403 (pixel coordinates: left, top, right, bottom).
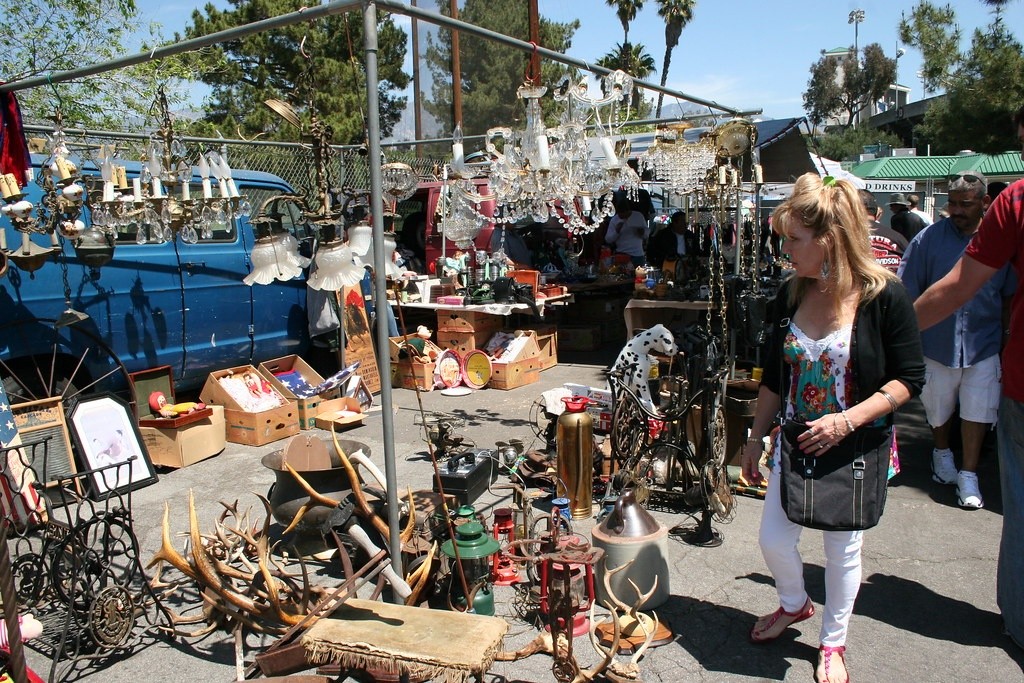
362, 176, 591, 282
0, 153, 377, 471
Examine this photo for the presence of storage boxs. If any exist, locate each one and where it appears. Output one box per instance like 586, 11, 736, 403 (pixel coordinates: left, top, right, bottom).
125, 299, 624, 469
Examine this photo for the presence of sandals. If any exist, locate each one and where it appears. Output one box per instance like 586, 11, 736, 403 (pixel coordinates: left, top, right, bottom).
815, 645, 849, 683
749, 596, 815, 644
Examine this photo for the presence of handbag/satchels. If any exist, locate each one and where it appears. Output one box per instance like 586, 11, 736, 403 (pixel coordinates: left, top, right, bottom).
780, 419, 893, 531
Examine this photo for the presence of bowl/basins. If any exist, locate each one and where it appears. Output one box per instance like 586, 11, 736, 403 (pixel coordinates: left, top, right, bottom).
437, 348, 463, 387
463, 349, 492, 389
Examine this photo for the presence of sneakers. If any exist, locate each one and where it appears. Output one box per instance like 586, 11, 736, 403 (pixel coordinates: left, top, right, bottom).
955, 469, 985, 508
931, 447, 958, 485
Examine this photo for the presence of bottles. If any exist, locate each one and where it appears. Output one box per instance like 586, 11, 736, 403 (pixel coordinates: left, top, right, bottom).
261, 440, 371, 533
556, 393, 592, 520
475, 269, 485, 282
491, 266, 498, 280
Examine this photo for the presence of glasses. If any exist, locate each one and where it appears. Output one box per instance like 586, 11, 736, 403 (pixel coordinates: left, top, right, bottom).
937, 213, 942, 218
950, 174, 986, 187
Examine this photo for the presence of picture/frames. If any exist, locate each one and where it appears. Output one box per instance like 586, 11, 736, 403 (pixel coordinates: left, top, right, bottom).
67, 390, 159, 501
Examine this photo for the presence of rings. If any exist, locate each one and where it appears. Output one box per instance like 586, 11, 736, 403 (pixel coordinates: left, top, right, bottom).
808, 430, 814, 435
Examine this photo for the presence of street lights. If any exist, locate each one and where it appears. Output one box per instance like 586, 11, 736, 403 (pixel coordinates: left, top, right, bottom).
846, 8, 866, 125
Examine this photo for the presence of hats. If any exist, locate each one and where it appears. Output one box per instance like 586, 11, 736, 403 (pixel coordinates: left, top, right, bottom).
935, 201, 951, 219
885, 193, 911, 206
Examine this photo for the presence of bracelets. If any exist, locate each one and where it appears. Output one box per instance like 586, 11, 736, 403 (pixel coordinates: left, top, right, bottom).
877, 388, 898, 412
746, 438, 764, 446
842, 409, 854, 432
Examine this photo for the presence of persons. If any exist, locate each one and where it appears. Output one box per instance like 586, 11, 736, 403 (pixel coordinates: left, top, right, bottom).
895, 170, 1024, 646
645, 209, 700, 270
858, 190, 909, 275
384, 215, 423, 272
605, 198, 650, 269
884, 193, 934, 243
492, 205, 533, 265
740, 171, 927, 683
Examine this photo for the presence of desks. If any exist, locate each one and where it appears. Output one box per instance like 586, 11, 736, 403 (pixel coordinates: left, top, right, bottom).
625, 298, 721, 343
386, 289, 574, 316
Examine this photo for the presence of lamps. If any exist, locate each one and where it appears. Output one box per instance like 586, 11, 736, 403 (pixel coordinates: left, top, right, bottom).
0, 71, 758, 295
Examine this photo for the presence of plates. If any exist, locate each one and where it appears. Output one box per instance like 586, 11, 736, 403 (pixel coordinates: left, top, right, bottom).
441, 387, 471, 396
